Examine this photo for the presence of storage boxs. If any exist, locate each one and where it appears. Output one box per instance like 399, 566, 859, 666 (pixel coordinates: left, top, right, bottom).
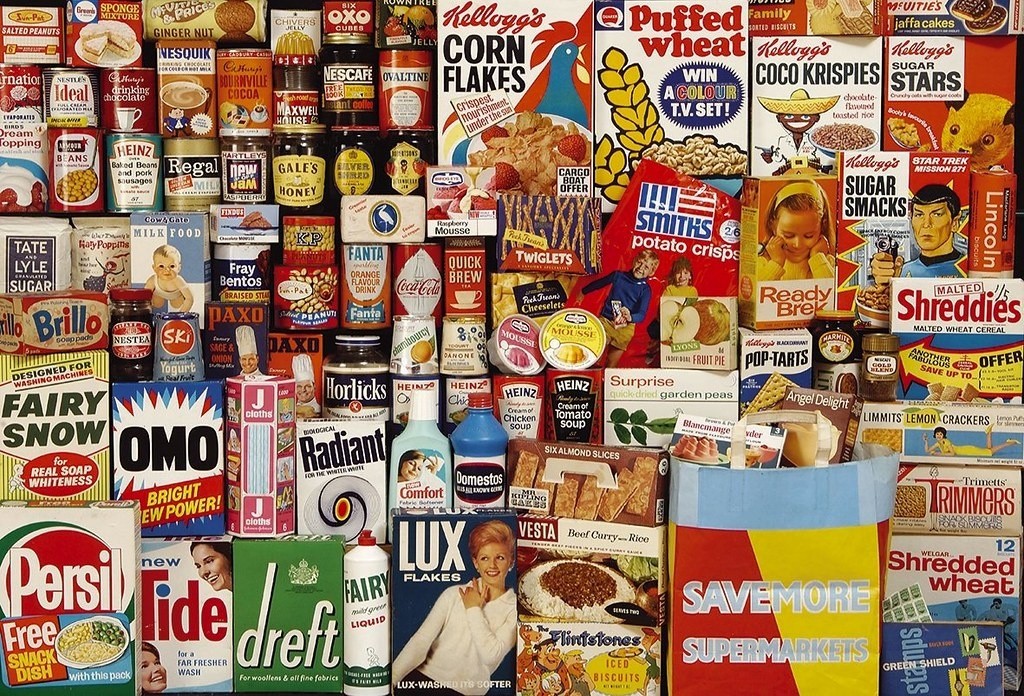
0, 0, 1024, 696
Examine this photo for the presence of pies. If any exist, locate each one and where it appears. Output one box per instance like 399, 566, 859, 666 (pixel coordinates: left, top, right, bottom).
511, 450, 656, 521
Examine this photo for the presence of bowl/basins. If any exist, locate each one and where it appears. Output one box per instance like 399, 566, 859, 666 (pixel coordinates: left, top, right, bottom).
486, 314, 547, 375
538, 308, 607, 370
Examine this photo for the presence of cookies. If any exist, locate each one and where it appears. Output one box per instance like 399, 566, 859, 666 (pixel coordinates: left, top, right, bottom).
217, 31, 258, 46
806, 0, 873, 35
952, 0, 1007, 33
894, 485, 927, 518
862, 428, 903, 454
742, 372, 800, 420
214, 0, 255, 33
492, 272, 578, 331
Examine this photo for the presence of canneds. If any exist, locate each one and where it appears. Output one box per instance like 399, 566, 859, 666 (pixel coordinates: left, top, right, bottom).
322, 335, 391, 425
109, 288, 155, 383
808, 308, 900, 402
390, 367, 604, 445
272, 32, 437, 216
0, 39, 273, 215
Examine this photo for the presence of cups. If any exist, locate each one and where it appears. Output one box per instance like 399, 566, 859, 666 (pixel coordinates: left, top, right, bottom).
455, 290, 482, 304
116, 107, 142, 129
159, 81, 213, 136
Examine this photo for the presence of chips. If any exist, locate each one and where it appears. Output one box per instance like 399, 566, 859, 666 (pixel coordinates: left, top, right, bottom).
468, 110, 591, 195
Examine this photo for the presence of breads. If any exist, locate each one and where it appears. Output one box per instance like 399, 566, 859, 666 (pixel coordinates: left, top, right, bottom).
79, 20, 136, 64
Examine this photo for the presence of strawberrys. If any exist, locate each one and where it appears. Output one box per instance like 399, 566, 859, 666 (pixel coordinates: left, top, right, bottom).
558, 135, 586, 161
481, 125, 510, 149
494, 162, 519, 191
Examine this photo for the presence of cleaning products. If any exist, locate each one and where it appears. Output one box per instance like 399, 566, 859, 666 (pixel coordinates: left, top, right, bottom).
388, 388, 455, 542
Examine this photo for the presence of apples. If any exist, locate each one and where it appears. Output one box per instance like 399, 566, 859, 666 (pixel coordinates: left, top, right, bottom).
660, 298, 731, 346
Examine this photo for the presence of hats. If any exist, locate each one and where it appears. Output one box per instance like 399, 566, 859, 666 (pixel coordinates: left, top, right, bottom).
291, 355, 315, 384
236, 325, 257, 355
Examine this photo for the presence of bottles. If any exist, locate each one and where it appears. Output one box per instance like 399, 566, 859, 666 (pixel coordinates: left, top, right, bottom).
451, 393, 509, 510
387, 389, 457, 544
342, 529, 390, 696
318, 32, 379, 129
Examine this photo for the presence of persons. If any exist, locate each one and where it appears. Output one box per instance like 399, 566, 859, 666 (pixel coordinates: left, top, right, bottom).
392, 521, 517, 696
190, 542, 233, 592
661, 257, 698, 296
142, 642, 167, 693
756, 179, 835, 279
144, 245, 194, 313
578, 250, 659, 368
923, 423, 1021, 457
398, 450, 443, 484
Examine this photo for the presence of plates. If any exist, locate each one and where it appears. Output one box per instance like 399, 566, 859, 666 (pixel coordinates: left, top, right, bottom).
111, 129, 143, 132
808, 125, 880, 158
74, 37, 142, 67
450, 303, 482, 309
668, 446, 729, 465
